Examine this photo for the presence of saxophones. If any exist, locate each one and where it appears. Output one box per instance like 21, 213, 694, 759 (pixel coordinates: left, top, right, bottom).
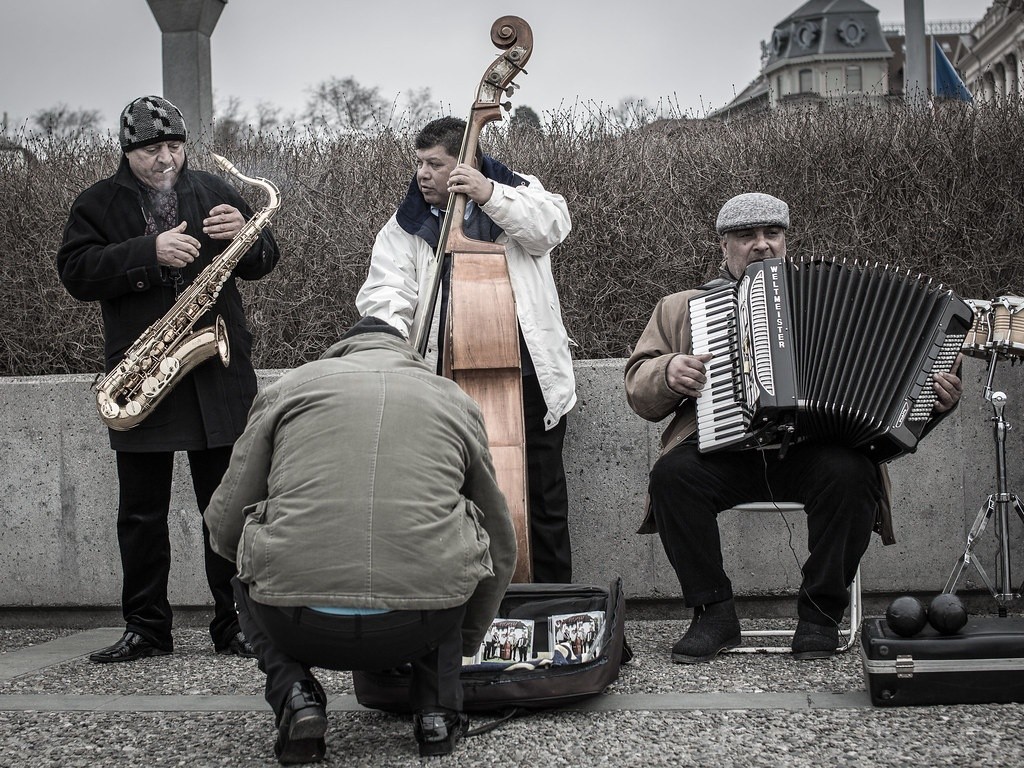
92, 154, 286, 431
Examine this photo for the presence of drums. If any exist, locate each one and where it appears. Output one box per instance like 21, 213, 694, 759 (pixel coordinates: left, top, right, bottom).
960, 299, 992, 359
992, 293, 1024, 355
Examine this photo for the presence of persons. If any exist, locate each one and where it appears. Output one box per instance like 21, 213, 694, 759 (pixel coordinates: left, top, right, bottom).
57, 96, 279, 662
624, 193, 964, 664
557, 624, 597, 660
483, 627, 529, 662
355, 116, 577, 583
203, 316, 518, 765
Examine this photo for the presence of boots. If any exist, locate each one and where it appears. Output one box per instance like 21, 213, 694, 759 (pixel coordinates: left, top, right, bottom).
792, 601, 839, 660
671, 596, 741, 663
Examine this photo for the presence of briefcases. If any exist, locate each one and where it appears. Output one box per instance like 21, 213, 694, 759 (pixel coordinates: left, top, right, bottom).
353, 577, 633, 738
860, 618, 1024, 708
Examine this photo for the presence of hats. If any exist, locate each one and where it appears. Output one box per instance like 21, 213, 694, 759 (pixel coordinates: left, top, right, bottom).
119, 96, 188, 152
716, 193, 790, 236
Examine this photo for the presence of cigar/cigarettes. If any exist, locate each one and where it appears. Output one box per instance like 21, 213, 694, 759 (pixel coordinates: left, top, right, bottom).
163, 166, 175, 175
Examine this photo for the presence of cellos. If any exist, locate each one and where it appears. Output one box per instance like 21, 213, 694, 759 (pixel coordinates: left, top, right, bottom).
414, 13, 535, 587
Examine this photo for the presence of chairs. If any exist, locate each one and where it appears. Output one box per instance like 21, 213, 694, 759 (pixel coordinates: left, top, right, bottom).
722, 500, 862, 654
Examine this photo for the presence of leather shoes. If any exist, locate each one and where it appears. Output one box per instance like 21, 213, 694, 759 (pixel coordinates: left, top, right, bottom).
414, 706, 469, 757
274, 682, 328, 762
89, 621, 174, 662
210, 619, 257, 657
